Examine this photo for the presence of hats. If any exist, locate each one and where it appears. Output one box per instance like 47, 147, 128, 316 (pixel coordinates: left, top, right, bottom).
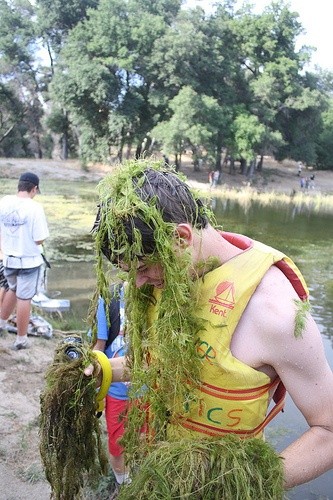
20, 173, 41, 195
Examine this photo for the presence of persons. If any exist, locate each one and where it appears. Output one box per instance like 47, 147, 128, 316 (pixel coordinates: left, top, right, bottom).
95, 167, 333, 491
209, 169, 221, 186
0, 260, 18, 334
300, 173, 315, 192
240, 157, 247, 176
0, 172, 50, 350
297, 160, 303, 178
87, 279, 158, 487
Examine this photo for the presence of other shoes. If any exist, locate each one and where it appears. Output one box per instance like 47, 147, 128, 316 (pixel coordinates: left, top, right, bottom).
16, 339, 32, 349
109, 455, 132, 485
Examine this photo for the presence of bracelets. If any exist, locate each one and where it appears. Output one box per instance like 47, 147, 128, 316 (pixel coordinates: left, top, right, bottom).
91, 350, 113, 419
